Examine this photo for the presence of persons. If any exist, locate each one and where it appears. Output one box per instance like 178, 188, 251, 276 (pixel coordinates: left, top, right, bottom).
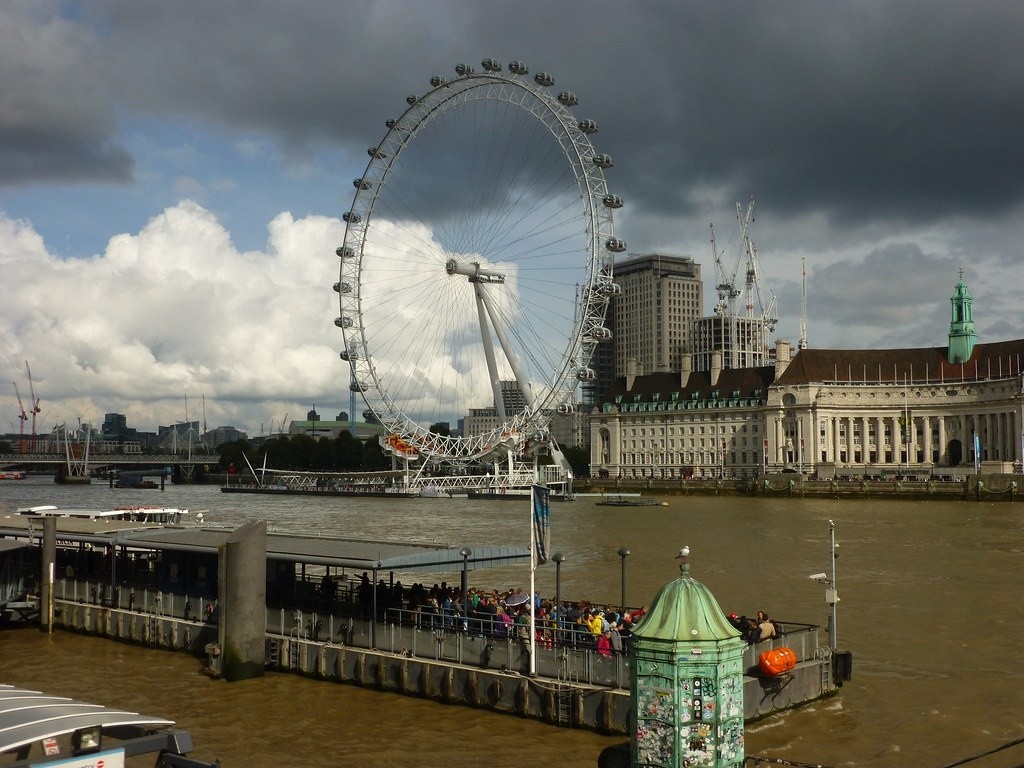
728, 611, 782, 646
354, 571, 649, 657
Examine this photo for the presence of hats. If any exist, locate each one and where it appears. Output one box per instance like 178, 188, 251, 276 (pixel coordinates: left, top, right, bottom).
730, 613, 736, 618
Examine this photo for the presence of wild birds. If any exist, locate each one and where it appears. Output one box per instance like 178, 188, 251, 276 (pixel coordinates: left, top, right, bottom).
674, 545, 690, 564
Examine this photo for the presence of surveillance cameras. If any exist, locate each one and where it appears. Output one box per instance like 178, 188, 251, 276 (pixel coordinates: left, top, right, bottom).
810, 573, 826, 579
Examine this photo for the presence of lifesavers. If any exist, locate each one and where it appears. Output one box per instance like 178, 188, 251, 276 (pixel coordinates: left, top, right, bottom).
501, 486, 506, 492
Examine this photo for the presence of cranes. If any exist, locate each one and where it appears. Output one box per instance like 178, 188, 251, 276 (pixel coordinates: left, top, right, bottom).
202, 394, 207, 434
710, 194, 808, 354
10, 381, 28, 452
25, 359, 42, 453
185, 392, 189, 422
259, 412, 289, 438
